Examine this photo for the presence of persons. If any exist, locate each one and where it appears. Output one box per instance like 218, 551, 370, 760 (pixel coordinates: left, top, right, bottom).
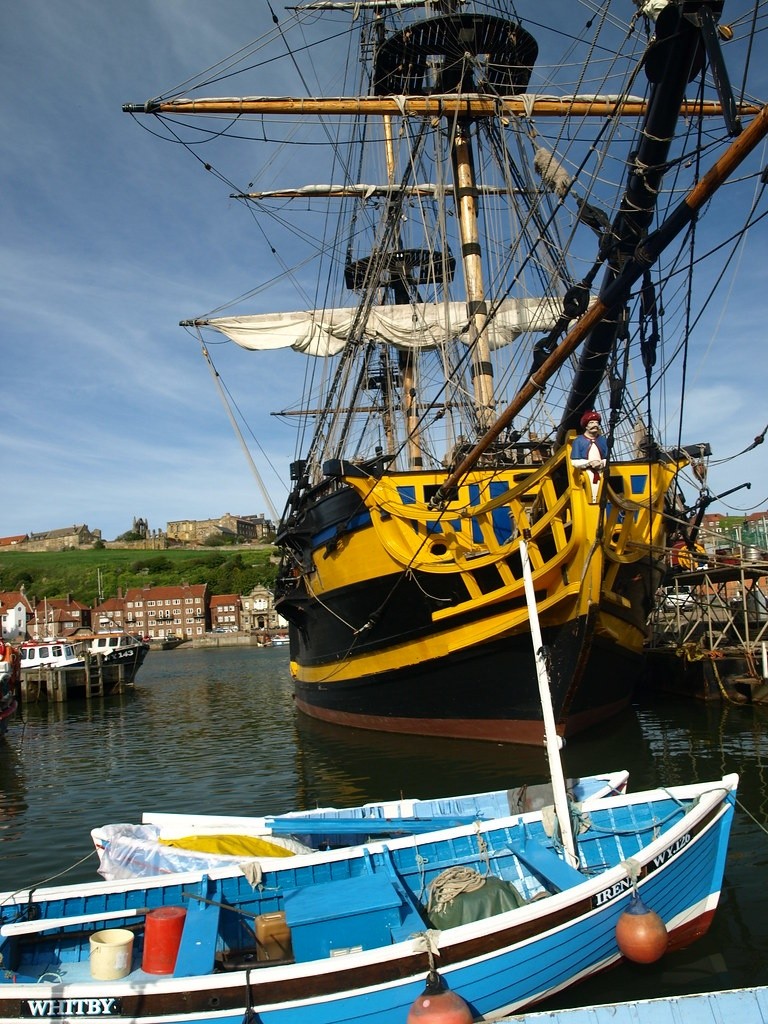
571, 412, 609, 504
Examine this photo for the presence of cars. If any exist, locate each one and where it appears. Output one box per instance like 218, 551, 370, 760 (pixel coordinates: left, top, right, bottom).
659, 586, 694, 613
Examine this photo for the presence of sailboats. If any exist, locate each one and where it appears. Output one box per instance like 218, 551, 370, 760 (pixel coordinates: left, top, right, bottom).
123, 2, 768, 756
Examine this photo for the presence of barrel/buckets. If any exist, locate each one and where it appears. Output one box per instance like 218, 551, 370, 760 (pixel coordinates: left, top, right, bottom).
88, 929, 134, 980
141, 906, 187, 974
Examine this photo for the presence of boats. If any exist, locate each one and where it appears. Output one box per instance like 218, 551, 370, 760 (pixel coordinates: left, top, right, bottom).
270, 635, 290, 648
0, 566, 192, 719
0, 770, 740, 1024
